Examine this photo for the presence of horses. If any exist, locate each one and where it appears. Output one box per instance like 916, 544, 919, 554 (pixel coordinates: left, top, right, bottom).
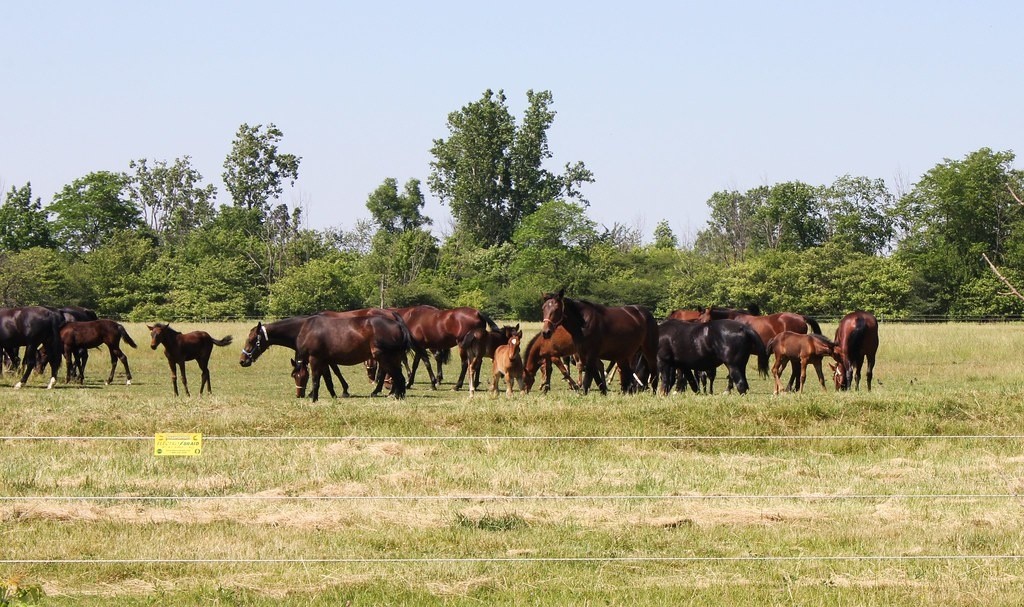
146, 322, 233, 397
238, 288, 880, 403
1, 305, 137, 389
